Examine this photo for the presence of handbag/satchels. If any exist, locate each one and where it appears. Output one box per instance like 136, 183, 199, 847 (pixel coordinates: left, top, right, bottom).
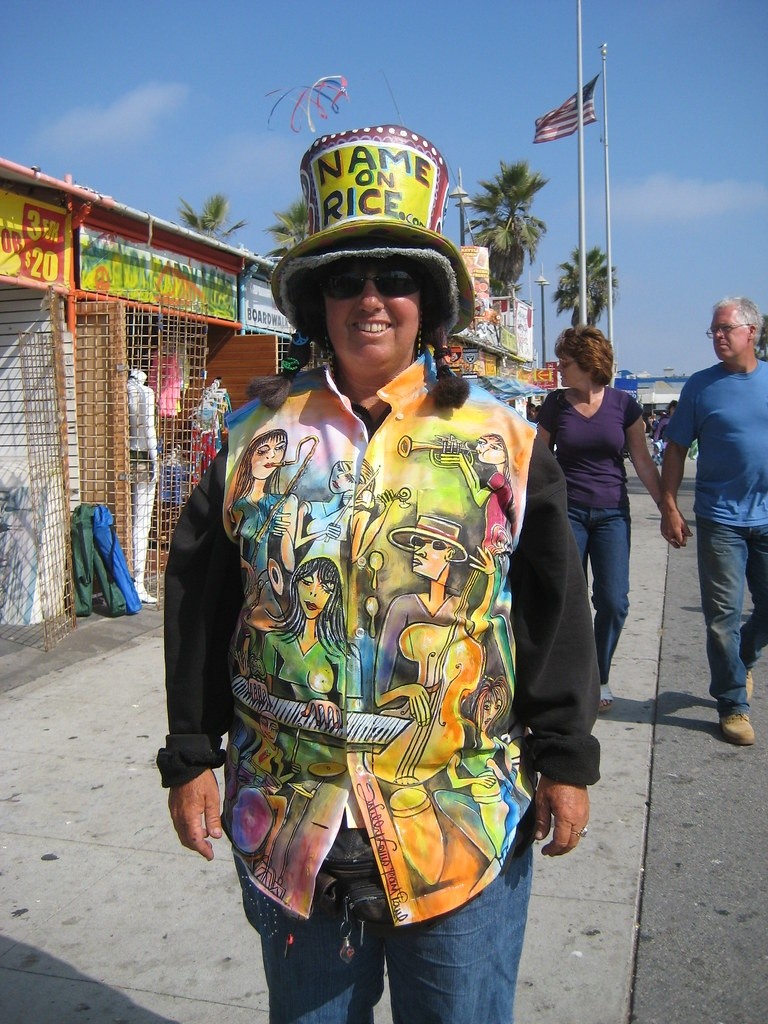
70, 503, 95, 617
93, 503, 142, 617
315, 829, 483, 934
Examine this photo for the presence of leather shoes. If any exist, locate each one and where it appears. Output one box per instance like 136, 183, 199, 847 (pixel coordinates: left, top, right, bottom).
719, 713, 755, 745
746, 667, 754, 702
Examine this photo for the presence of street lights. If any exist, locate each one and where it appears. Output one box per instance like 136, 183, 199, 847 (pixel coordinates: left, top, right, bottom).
535, 276, 550, 367
448, 185, 474, 246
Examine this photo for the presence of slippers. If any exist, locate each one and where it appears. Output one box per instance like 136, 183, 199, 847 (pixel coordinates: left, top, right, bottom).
598, 683, 613, 715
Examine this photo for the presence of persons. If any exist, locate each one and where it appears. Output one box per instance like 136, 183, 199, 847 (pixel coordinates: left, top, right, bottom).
118, 371, 155, 605
156, 123, 600, 1024
529, 323, 653, 711
526, 403, 542, 422
660, 295, 768, 746
642, 400, 699, 465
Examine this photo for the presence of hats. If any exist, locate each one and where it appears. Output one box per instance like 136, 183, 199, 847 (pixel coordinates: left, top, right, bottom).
270, 125, 475, 337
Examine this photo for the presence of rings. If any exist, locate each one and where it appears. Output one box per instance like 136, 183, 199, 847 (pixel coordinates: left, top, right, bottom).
569, 826, 589, 841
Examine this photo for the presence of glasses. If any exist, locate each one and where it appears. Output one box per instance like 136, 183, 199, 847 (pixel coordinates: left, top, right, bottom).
319, 264, 424, 299
706, 323, 753, 338
559, 358, 577, 368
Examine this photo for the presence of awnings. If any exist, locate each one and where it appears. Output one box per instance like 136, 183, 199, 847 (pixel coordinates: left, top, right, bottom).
478, 375, 548, 402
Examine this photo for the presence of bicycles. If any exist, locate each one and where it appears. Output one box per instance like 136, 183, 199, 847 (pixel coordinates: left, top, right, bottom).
623, 450, 634, 463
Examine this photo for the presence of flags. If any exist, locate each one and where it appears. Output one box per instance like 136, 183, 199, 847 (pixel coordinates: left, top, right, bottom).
526, 73, 600, 145
